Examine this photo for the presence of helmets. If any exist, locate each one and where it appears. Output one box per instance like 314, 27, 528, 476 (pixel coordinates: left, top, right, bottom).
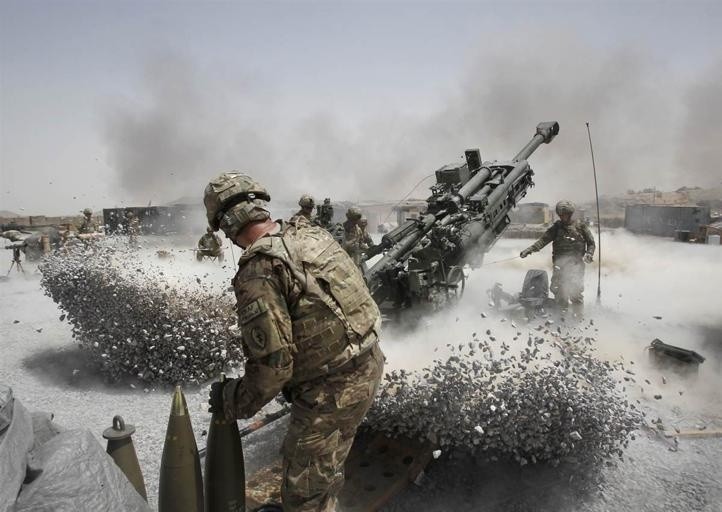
299, 193, 315, 208
556, 200, 575, 213
203, 170, 271, 232
345, 206, 368, 226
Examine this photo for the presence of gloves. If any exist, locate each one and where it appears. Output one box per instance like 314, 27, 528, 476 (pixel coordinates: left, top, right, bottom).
208, 379, 229, 412
520, 248, 531, 258
584, 252, 594, 264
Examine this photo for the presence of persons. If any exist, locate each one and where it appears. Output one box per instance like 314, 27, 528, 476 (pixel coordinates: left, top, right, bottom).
124, 209, 142, 237
193, 225, 226, 265
200, 168, 389, 512
73, 208, 103, 253
517, 196, 598, 323
289, 189, 374, 280
